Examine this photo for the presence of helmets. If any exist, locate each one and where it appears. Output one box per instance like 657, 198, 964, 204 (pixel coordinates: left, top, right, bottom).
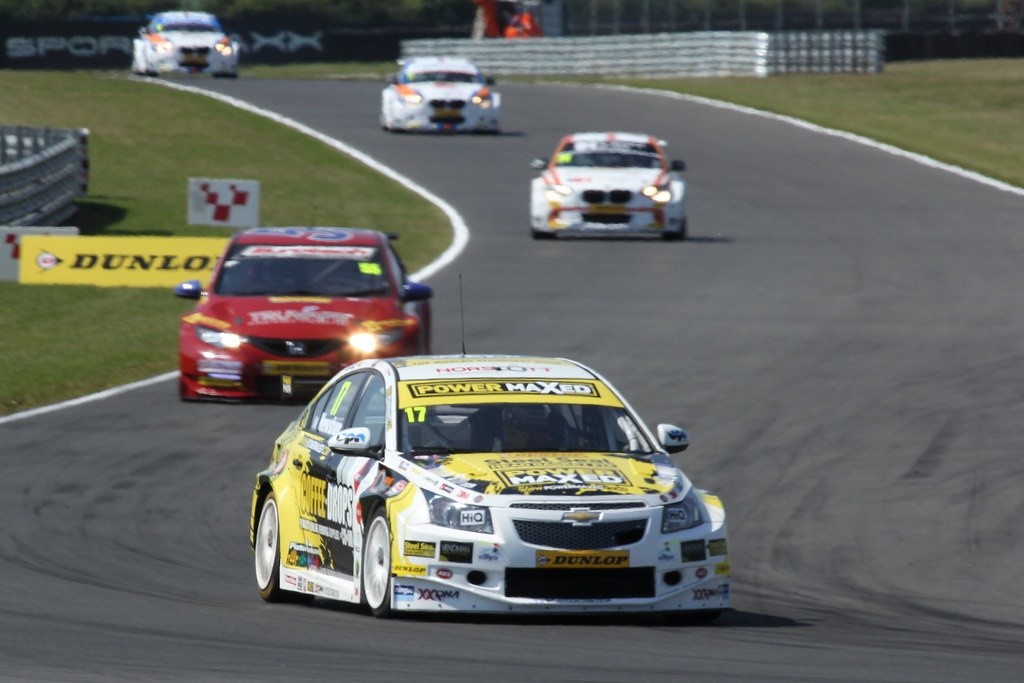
501, 403, 550, 445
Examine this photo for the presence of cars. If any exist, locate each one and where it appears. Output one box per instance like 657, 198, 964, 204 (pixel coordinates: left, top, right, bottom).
248, 354, 733, 623
131, 10, 240, 79
174, 226, 433, 403
380, 55, 502, 135
529, 131, 688, 241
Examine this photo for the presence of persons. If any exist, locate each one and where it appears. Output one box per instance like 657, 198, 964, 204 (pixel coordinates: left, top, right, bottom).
503, 5, 542, 37
489, 404, 565, 453
